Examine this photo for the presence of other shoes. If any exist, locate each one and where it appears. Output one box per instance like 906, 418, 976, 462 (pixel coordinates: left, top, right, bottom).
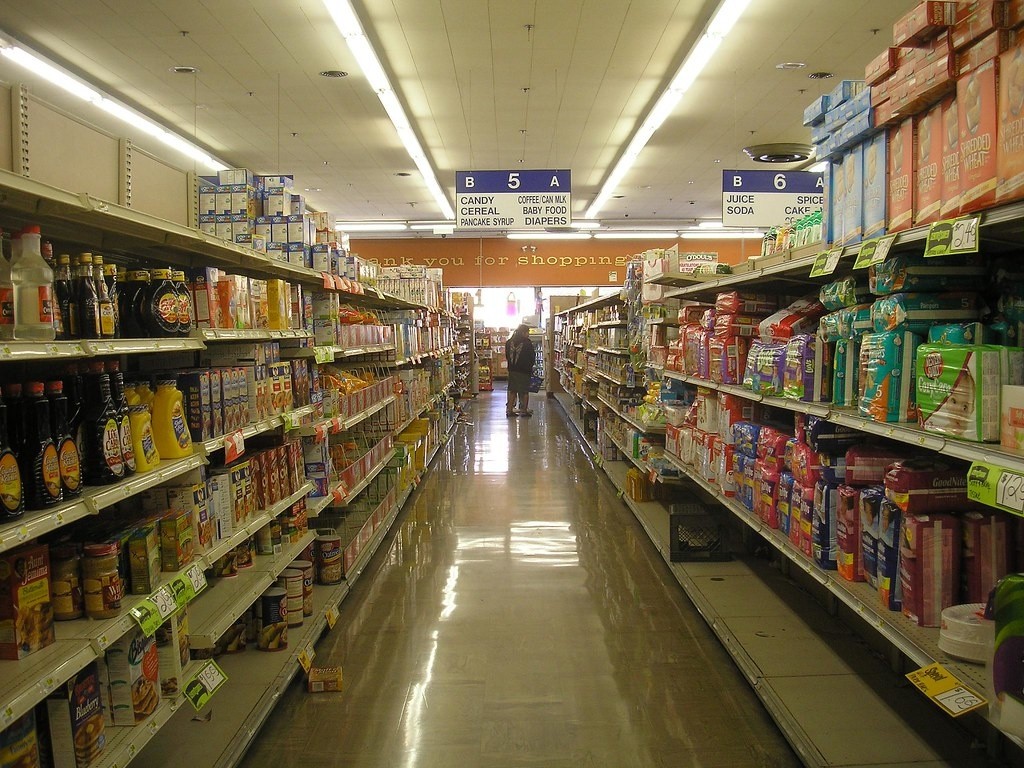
506, 412, 520, 418
526, 408, 533, 412
521, 412, 532, 417
513, 407, 520, 412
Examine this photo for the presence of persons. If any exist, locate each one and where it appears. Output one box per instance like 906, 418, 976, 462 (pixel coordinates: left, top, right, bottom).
880, 508, 893, 533
864, 502, 873, 526
814, 489, 825, 521
943, 365, 975, 436
505, 324, 536, 417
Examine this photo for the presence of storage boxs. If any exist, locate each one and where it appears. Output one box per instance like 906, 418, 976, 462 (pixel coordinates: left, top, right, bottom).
360, 418, 431, 502
188, 168, 442, 346
0, 342, 336, 768
670, 503, 739, 562
804, 0, 1024, 252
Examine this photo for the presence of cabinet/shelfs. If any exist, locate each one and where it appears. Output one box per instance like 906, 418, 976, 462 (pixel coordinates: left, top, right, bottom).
335, 289, 470, 508
545, 226, 1024, 767
330, 275, 400, 589
0, 172, 346, 768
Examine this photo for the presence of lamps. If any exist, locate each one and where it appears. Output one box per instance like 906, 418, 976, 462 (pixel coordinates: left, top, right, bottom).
503, 0, 765, 239
742, 142, 815, 163
322, 0, 464, 231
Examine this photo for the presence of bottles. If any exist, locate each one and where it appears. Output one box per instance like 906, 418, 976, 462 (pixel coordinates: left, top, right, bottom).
0, 359, 192, 524
761, 211, 822, 256
0, 225, 191, 341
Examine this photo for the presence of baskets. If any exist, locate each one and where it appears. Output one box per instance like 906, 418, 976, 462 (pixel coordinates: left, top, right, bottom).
528, 372, 544, 394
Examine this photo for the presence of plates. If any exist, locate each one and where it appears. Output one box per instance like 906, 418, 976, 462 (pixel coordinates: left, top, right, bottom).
939, 603, 995, 665
